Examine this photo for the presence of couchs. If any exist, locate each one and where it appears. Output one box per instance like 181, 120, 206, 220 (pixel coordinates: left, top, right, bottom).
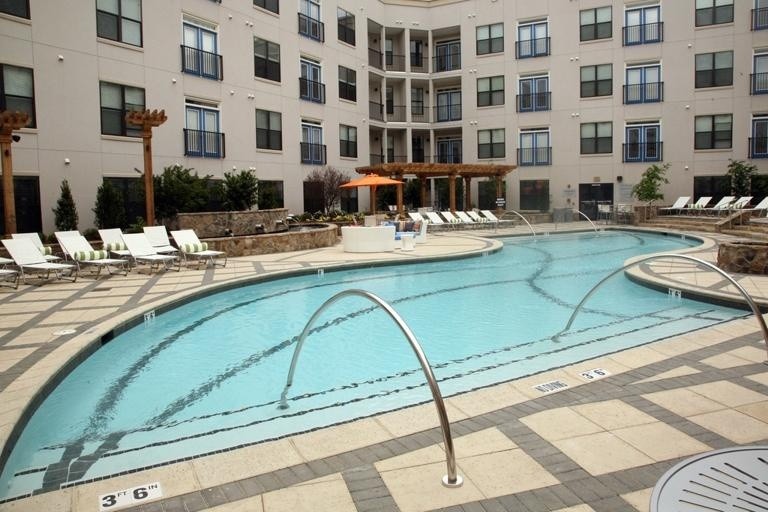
340, 220, 428, 253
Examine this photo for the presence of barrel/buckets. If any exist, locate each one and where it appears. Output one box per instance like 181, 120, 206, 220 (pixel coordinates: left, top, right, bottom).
401, 234, 414, 251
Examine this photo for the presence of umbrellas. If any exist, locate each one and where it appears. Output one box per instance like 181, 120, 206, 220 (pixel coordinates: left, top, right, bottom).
338, 172, 406, 215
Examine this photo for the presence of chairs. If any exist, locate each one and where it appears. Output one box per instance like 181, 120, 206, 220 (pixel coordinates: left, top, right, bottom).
658, 196, 768, 219
0, 225, 228, 290
595, 204, 637, 224
408, 206, 518, 233
